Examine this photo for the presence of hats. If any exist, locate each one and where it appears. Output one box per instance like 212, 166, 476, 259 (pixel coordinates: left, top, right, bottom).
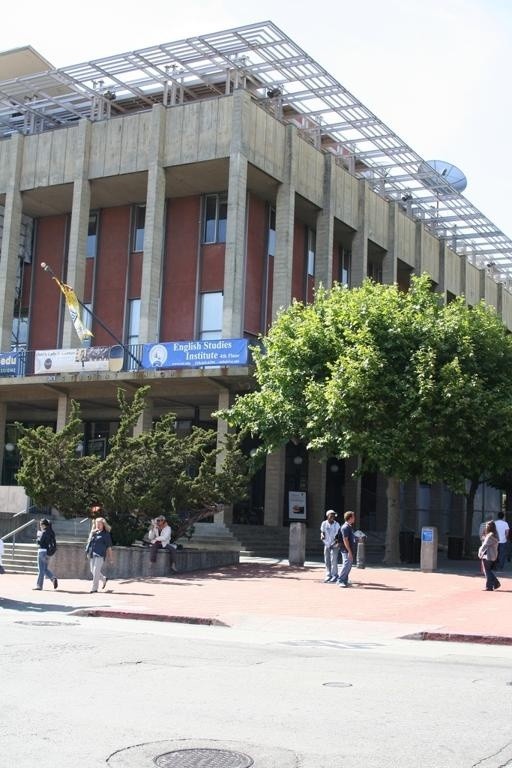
325, 510, 336, 516
156, 515, 166, 520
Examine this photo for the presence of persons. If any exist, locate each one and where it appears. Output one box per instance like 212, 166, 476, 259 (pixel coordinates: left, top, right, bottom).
83, 516, 113, 592
149, 515, 177, 572
477, 520, 501, 590
320, 509, 341, 583
479, 515, 494, 576
494, 510, 510, 572
0, 537, 6, 574
335, 510, 357, 587
33, 519, 58, 589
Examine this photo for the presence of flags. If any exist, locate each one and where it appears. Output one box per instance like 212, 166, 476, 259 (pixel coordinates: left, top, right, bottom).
51, 276, 95, 343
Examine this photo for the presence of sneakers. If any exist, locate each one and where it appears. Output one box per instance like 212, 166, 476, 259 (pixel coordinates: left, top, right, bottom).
329, 575, 338, 582
33, 587, 43, 592
90, 589, 97, 596
171, 564, 178, 571
346, 582, 352, 587
494, 583, 500, 590
52, 577, 58, 589
149, 562, 154, 569
482, 588, 494, 591
324, 577, 331, 583
338, 581, 347, 588
102, 577, 107, 590
